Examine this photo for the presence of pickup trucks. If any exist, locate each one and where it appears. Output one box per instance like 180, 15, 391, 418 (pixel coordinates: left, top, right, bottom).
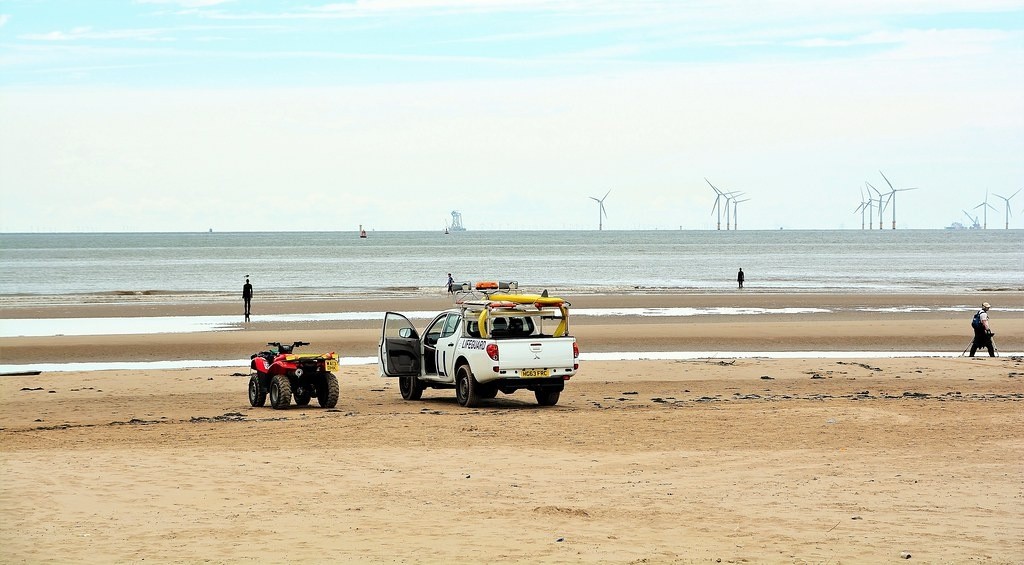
378, 281, 580, 407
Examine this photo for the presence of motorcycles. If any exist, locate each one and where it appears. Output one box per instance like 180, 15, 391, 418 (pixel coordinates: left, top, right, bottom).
248, 340, 340, 411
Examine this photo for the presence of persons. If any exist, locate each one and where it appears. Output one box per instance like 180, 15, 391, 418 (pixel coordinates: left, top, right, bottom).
242, 279, 253, 315
737, 268, 744, 289
969, 302, 995, 358
444, 273, 455, 298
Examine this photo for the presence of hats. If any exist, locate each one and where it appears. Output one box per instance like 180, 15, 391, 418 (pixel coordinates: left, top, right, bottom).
983, 303, 990, 308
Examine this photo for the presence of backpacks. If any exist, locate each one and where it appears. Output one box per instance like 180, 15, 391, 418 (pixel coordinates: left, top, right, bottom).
971, 310, 985, 330
